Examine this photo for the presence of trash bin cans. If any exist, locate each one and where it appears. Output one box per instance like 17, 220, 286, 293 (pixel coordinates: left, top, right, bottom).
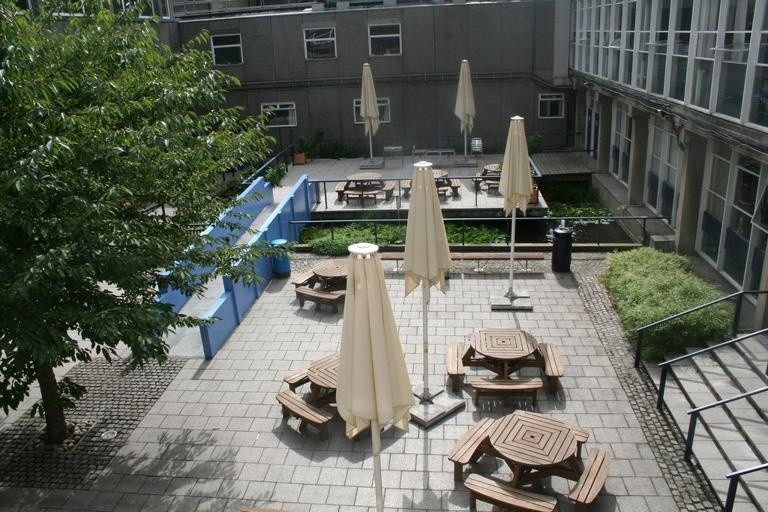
271, 239, 291, 277
295, 152, 305, 165
552, 228, 572, 272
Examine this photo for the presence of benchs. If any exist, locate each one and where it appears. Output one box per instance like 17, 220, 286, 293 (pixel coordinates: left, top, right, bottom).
335, 180, 395, 207
410, 144, 455, 166
446, 409, 614, 512
382, 146, 404, 168
380, 252, 544, 272
474, 173, 500, 196
276, 372, 334, 443
403, 179, 461, 201
448, 338, 565, 412
292, 271, 345, 314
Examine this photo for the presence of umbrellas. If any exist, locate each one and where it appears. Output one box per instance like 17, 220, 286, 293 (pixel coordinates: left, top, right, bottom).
334, 242, 416, 511
402, 161, 454, 393
453, 59, 476, 158
498, 115, 533, 290
360, 63, 380, 160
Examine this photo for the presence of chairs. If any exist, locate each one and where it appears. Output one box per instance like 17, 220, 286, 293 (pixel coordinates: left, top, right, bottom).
470, 137, 483, 159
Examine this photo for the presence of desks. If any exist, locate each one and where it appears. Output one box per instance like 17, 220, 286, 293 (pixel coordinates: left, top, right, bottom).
346, 172, 386, 200
431, 169, 452, 187
481, 164, 503, 180
462, 327, 546, 379
313, 262, 350, 290
308, 352, 340, 409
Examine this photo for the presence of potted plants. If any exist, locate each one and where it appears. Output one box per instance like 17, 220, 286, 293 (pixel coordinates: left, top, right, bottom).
293, 141, 307, 164
